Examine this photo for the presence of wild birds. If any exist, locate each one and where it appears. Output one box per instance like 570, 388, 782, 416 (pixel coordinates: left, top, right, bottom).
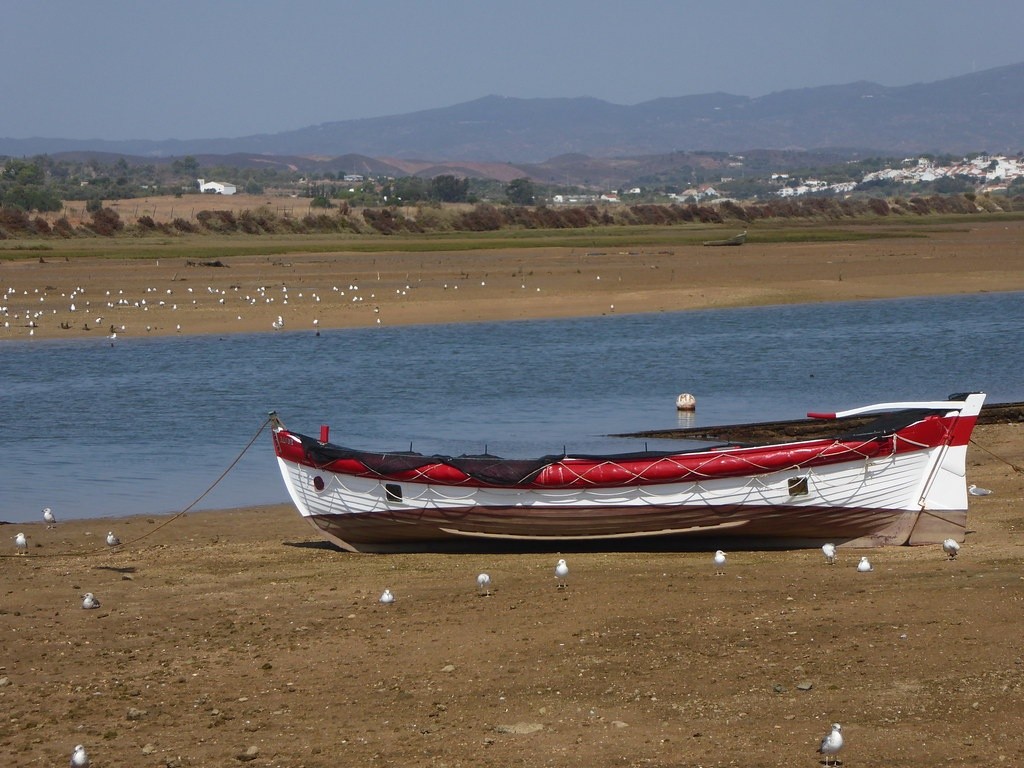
13, 532, 27, 555
943, 538, 961, 561
1, 263, 632, 347
815, 723, 843, 768
967, 485, 993, 496
856, 557, 874, 572
378, 589, 396, 602
41, 507, 56, 529
82, 592, 101, 609
106, 532, 122, 554
554, 559, 569, 589
713, 550, 728, 576
70, 744, 89, 768
821, 542, 837, 565
476, 574, 491, 597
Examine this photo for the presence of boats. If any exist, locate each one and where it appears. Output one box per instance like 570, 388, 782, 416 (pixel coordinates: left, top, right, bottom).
271, 390, 987, 550
702, 230, 748, 246
